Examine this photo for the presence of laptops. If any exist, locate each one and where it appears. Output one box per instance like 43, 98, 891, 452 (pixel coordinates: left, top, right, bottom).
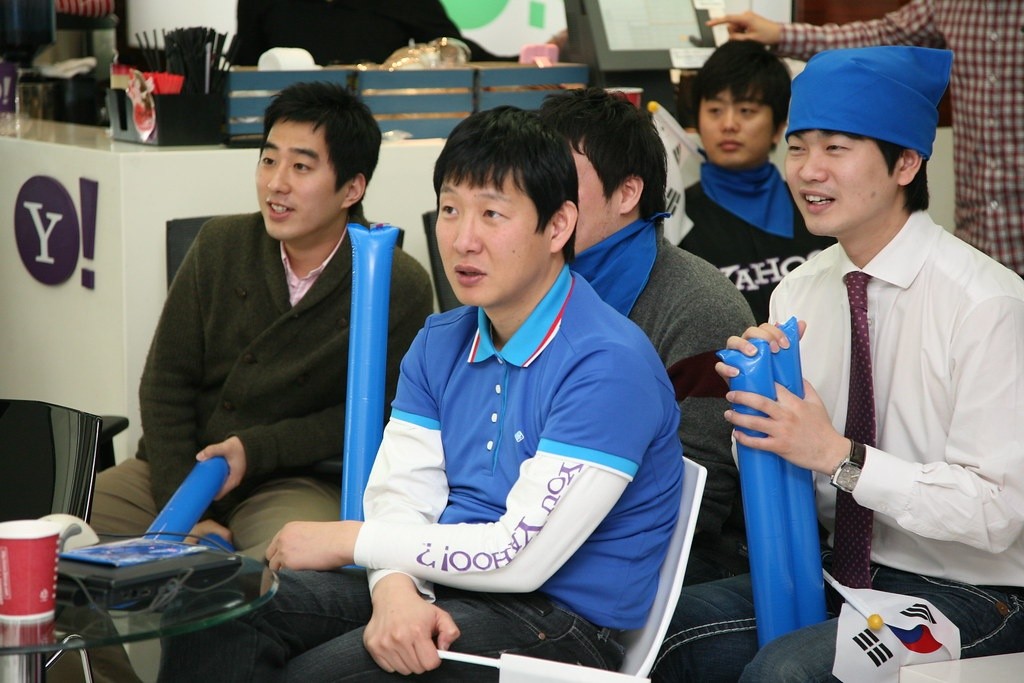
55, 549, 242, 608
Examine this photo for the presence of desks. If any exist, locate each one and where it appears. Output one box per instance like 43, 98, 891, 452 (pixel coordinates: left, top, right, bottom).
0, 555, 283, 683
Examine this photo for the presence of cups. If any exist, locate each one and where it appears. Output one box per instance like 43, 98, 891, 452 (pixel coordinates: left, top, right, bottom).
13, 80, 46, 138
259, 48, 316, 71
0, 519, 60, 623
606, 88, 644, 108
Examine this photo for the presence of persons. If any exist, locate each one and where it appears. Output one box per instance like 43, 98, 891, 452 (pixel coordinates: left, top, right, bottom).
41, 81, 436, 683
650, 50, 1024, 683
154, 103, 684, 683
543, 85, 758, 583
677, 39, 838, 326
704, 0, 1024, 276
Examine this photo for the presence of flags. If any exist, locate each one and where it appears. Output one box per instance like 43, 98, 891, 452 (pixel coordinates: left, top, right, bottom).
500, 652, 650, 683
832, 580, 961, 683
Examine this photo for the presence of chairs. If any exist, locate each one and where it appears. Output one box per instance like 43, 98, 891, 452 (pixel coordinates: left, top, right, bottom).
0, 398, 104, 683
437, 455, 709, 683
95, 199, 363, 470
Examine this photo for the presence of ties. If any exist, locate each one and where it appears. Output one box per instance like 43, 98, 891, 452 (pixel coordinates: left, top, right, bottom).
828, 271, 876, 617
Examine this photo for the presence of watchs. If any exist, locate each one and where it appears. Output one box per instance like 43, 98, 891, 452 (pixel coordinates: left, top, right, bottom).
829, 439, 866, 495
